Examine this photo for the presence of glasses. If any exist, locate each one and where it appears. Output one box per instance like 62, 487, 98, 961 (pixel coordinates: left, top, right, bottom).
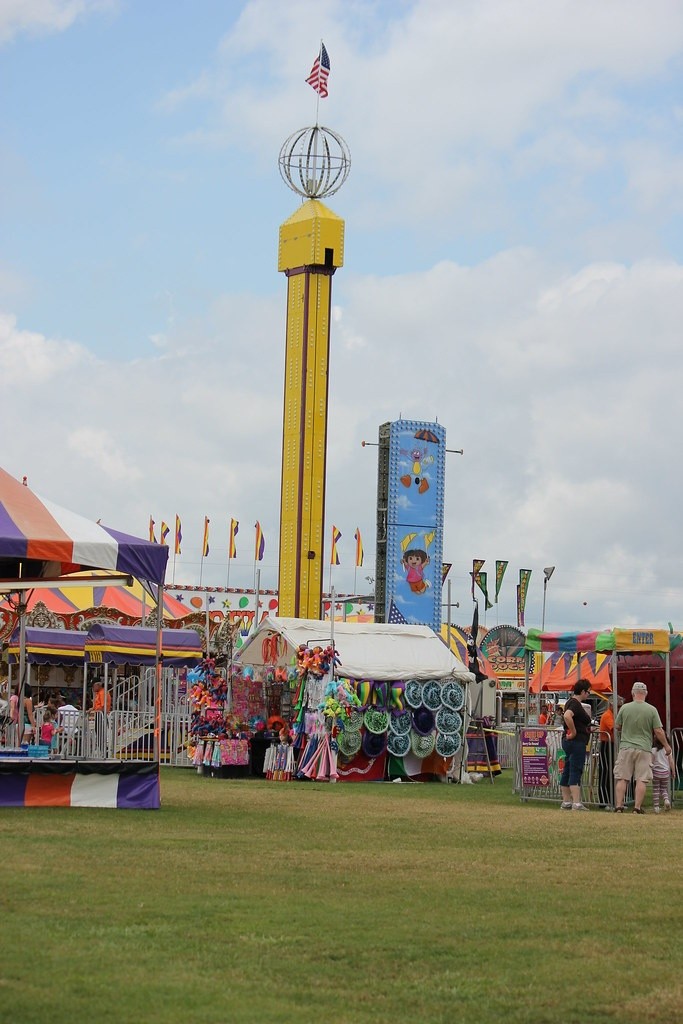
584, 688, 591, 695
618, 705, 624, 708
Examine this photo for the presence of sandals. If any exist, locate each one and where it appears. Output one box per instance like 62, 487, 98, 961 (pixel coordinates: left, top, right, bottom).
633, 807, 644, 814
615, 806, 624, 812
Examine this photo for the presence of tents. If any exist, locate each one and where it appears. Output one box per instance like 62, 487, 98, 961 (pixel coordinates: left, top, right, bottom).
0, 467, 170, 762
8, 625, 120, 756
325, 613, 496, 678
82, 623, 204, 760
529, 651, 612, 701
227, 616, 476, 784
524, 628, 683, 806
0, 569, 195, 620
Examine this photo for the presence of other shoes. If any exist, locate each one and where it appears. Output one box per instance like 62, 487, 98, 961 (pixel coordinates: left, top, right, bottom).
572, 802, 590, 811
664, 800, 672, 811
654, 808, 661, 815
560, 801, 574, 809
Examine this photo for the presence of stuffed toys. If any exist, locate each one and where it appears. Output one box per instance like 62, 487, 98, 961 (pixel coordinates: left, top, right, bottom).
318, 680, 361, 721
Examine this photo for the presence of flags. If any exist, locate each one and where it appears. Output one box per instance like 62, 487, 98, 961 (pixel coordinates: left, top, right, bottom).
150, 514, 364, 566
305, 42, 330, 98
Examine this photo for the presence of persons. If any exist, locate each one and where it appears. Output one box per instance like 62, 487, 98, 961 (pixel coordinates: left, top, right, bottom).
560, 679, 592, 810
652, 730, 675, 814
613, 682, 672, 814
279, 727, 290, 747
189, 658, 227, 768
539, 705, 598, 765
295, 644, 342, 681
10, 677, 111, 755
598, 695, 629, 811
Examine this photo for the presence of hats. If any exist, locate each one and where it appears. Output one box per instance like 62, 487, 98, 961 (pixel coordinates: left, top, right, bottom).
336, 679, 465, 759
89, 676, 101, 687
632, 681, 647, 690
